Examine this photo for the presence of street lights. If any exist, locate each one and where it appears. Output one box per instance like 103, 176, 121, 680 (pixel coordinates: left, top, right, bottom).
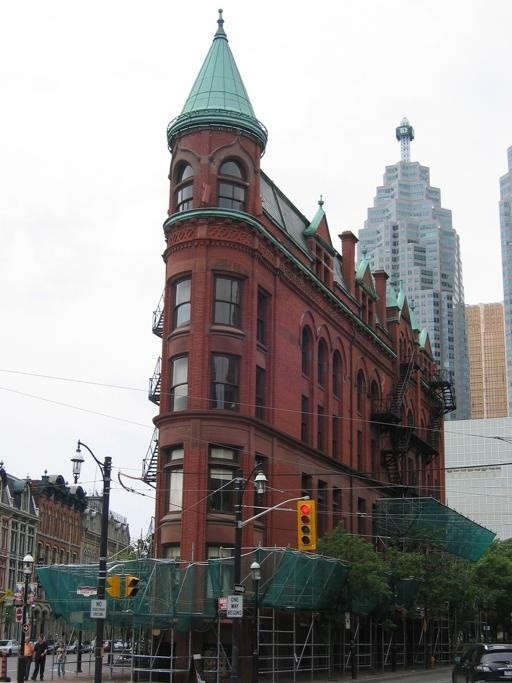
250, 561, 262, 682
16, 554, 34, 682
71, 437, 112, 683
229, 462, 269, 682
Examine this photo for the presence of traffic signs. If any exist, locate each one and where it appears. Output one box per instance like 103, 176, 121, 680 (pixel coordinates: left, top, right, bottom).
227, 596, 244, 620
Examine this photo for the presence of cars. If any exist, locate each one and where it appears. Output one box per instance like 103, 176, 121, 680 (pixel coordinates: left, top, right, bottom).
47, 638, 151, 655
452, 643, 512, 682
0, 639, 21, 656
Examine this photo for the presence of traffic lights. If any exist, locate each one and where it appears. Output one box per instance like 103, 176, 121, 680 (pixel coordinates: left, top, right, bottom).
125, 575, 139, 597
105, 575, 120, 598
297, 500, 318, 552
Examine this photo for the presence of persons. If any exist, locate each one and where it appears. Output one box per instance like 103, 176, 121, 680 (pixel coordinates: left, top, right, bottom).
23, 636, 35, 680
30, 633, 49, 681
55, 640, 67, 679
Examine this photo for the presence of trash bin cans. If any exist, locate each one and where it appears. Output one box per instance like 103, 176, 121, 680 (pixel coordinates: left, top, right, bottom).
453, 656, 461, 665
